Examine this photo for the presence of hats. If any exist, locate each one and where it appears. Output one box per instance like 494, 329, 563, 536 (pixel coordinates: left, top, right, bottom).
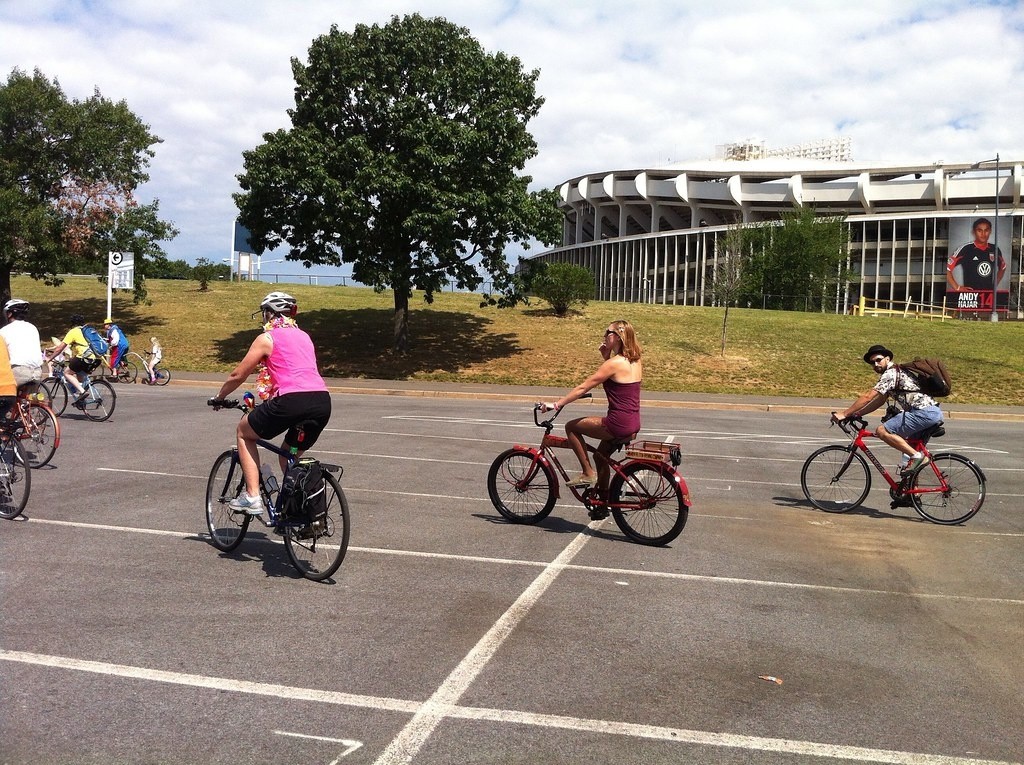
103, 318, 114, 323
864, 344, 893, 363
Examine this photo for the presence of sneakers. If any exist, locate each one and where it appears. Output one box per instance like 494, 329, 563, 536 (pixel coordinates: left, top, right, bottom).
229, 491, 264, 515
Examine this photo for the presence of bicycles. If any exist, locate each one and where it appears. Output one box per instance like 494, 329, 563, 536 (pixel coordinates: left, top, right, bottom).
37, 357, 116, 422
486, 391, 692, 545
800, 409, 988, 526
0, 380, 61, 520
56, 354, 170, 386
206, 400, 350, 579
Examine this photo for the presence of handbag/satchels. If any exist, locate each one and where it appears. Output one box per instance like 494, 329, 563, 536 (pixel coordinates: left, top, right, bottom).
281, 459, 329, 522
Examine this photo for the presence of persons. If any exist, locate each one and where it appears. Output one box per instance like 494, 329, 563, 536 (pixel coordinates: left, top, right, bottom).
832, 345, 943, 508
539, 320, 643, 516
0, 298, 163, 504
946, 217, 1007, 292
210, 292, 332, 535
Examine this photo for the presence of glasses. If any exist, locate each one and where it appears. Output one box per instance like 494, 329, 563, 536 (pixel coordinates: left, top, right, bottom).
606, 329, 615, 335
869, 356, 885, 364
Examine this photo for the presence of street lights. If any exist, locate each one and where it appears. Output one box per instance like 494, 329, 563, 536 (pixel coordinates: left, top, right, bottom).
223, 258, 283, 281
972, 159, 1000, 322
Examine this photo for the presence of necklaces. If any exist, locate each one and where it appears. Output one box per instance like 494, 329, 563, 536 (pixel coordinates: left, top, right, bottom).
257, 318, 298, 401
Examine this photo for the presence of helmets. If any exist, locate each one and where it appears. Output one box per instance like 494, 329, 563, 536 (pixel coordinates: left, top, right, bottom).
261, 291, 299, 316
4, 298, 30, 313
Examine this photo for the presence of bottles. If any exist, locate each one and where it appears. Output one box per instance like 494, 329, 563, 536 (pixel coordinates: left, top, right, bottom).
260, 463, 278, 495
26, 393, 44, 401
69, 383, 76, 393
899, 453, 910, 470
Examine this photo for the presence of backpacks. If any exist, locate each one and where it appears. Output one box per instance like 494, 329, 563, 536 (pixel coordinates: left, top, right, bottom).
81, 325, 108, 357
890, 358, 951, 397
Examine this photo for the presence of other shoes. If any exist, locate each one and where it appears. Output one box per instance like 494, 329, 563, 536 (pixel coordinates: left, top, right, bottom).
901, 453, 931, 475
74, 390, 89, 403
889, 496, 923, 507
565, 473, 597, 487
151, 376, 157, 384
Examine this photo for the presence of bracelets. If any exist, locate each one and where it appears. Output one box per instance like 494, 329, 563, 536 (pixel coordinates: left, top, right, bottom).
553, 401, 558, 411
955, 285, 961, 290
